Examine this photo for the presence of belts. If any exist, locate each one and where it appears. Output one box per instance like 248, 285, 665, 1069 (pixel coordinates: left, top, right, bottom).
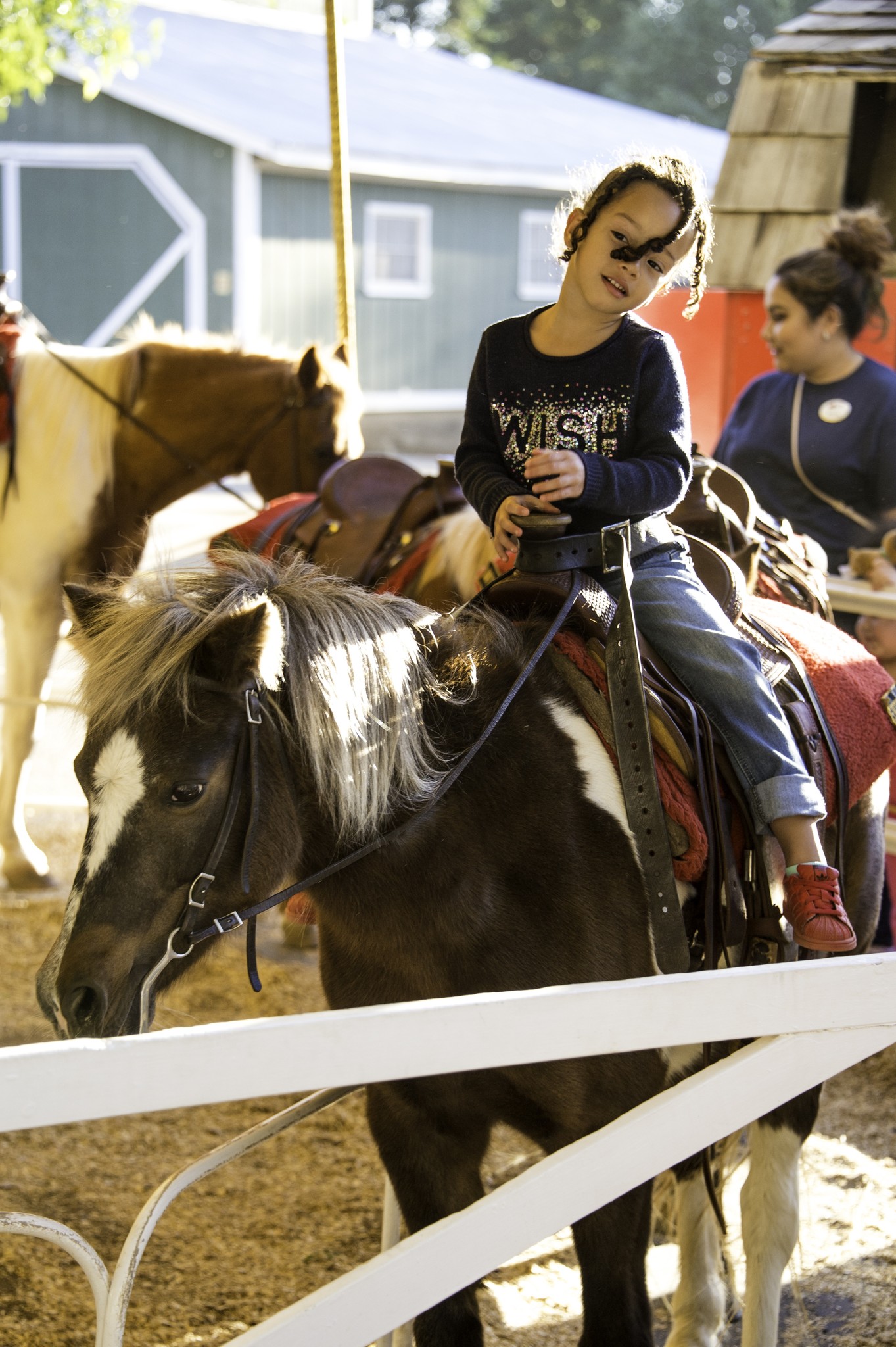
512, 510, 692, 977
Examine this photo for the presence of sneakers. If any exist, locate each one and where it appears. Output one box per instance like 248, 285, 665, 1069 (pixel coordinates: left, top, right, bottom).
781, 861, 858, 952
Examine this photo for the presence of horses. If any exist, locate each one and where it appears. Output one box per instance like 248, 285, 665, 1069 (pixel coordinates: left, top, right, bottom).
211, 452, 496, 617
1, 320, 366, 890
32, 530, 894, 1345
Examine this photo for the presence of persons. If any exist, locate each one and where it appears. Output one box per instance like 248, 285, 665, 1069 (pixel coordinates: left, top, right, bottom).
712, 204, 896, 685
454, 158, 857, 953
